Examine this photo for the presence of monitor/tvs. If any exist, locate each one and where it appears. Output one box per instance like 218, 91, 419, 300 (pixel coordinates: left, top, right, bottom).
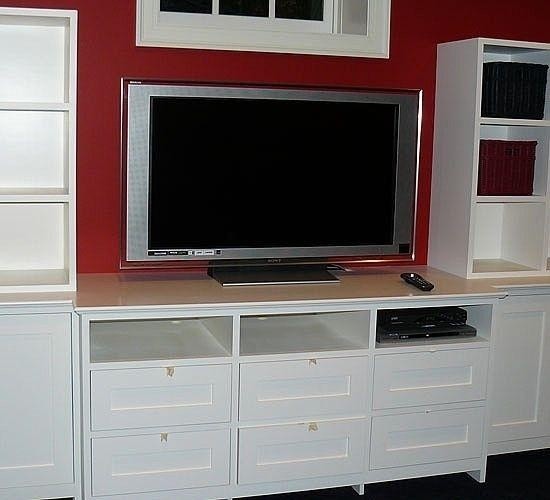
119, 75, 424, 287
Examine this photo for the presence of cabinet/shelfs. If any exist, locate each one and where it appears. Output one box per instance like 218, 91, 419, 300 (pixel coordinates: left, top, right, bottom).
2, 6, 80, 296
426, 37, 549, 280
235, 309, 371, 488
81, 313, 234, 498
368, 303, 495, 471
2, 312, 76, 490
486, 294, 549, 446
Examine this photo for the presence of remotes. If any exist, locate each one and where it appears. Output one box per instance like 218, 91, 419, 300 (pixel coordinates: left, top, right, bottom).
401, 272, 434, 292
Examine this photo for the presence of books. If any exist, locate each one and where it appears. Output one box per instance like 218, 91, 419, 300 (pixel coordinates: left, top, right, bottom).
477, 62, 548, 196
